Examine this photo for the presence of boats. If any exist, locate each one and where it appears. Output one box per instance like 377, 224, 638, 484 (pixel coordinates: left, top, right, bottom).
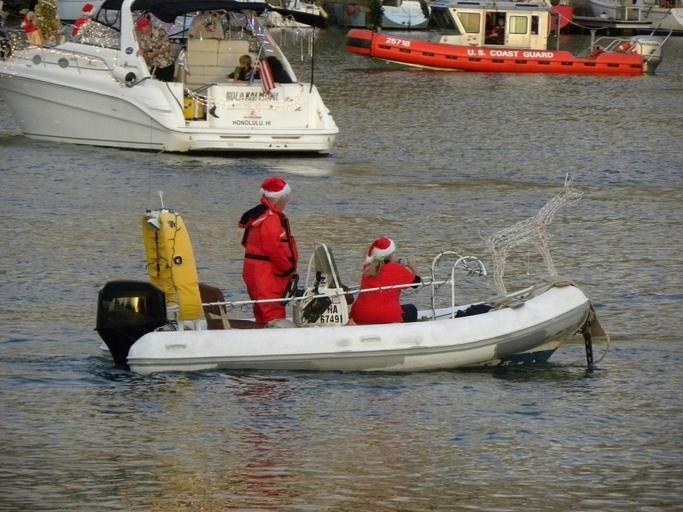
99, 250, 597, 377
345, 3, 664, 79
0, 2, 340, 157
425, 0, 681, 37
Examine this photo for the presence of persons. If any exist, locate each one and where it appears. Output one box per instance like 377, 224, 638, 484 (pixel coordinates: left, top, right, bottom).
134, 18, 175, 82
486, 18, 503, 45
236, 178, 298, 325
350, 236, 420, 324
227, 53, 294, 83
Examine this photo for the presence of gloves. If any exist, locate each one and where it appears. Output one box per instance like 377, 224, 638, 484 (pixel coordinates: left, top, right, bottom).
302, 293, 331, 322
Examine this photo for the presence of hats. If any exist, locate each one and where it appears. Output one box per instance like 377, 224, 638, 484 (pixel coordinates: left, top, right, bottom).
367, 237, 396, 265
260, 178, 292, 199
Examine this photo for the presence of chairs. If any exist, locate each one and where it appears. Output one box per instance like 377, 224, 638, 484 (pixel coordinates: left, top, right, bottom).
199, 281, 267, 329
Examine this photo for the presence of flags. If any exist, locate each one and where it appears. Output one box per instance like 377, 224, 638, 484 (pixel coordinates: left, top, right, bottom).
254, 55, 276, 95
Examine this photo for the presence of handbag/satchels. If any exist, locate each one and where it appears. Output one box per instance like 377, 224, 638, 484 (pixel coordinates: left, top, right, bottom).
292, 243, 350, 326
